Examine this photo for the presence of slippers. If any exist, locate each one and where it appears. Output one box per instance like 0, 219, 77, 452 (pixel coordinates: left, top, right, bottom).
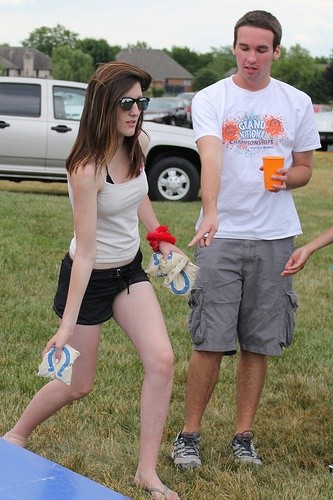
145, 486, 181, 500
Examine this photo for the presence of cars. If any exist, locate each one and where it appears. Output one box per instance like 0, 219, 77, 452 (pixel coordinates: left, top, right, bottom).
0, 76, 202, 201
57, 91, 333, 151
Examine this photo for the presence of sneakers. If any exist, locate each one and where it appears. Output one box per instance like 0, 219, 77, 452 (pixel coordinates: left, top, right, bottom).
171, 430, 203, 472
226, 430, 262, 465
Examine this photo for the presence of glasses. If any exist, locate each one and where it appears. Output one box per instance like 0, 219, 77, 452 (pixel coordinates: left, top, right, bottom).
119, 97, 150, 111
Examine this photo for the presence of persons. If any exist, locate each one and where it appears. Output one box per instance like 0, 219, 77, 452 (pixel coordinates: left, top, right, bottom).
0, 60, 201, 500
170, 9, 332, 472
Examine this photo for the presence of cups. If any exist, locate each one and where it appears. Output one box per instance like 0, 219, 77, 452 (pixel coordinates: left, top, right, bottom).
263, 156, 284, 190
